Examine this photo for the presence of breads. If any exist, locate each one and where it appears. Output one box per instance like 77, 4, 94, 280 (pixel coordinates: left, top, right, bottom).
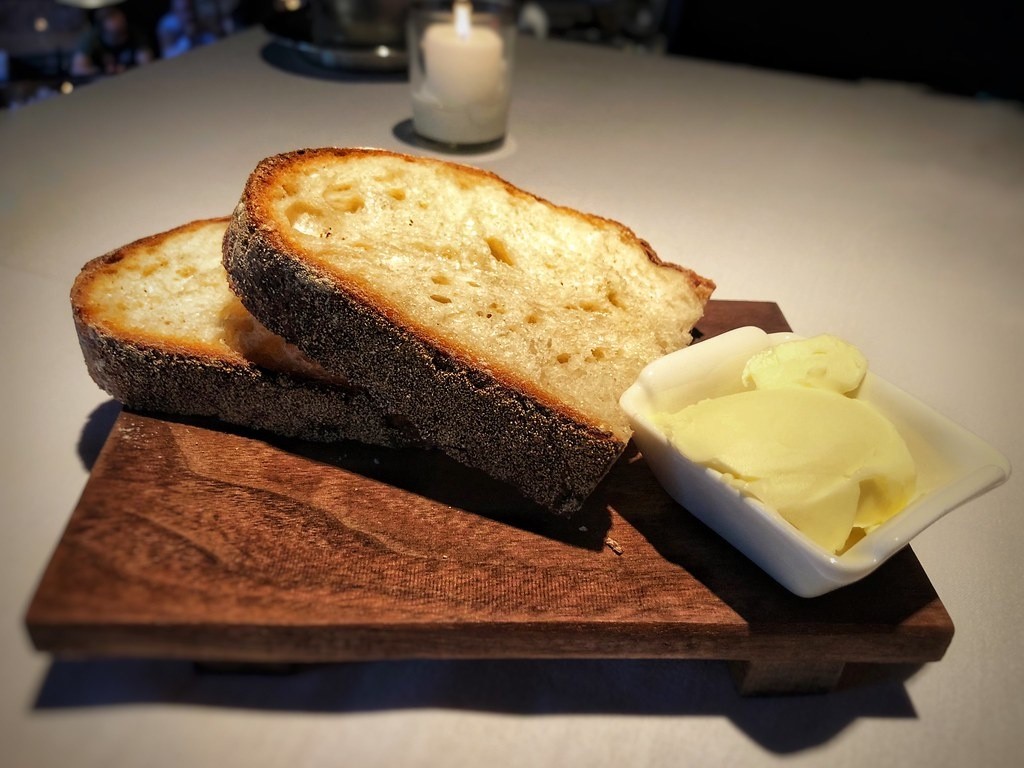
72, 216, 428, 452
224, 146, 717, 515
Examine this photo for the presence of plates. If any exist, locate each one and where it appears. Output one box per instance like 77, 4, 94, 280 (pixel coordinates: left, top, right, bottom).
620, 325, 1013, 599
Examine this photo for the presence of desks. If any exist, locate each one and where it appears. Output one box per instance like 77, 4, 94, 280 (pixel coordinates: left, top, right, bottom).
0, 26, 1024, 768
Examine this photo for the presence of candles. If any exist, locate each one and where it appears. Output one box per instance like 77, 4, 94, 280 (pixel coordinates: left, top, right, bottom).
422, 0, 507, 110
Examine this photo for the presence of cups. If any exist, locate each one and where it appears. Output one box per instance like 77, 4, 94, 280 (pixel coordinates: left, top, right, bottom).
406, 0, 518, 151
